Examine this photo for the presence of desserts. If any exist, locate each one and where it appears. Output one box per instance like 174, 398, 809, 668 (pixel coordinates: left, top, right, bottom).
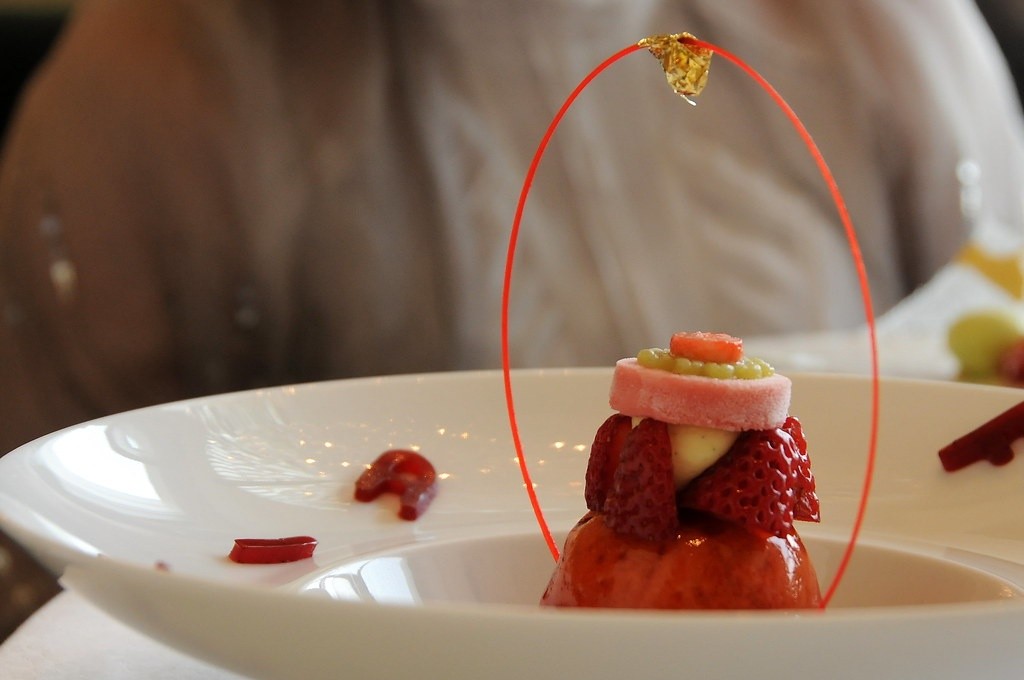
501, 29, 878, 609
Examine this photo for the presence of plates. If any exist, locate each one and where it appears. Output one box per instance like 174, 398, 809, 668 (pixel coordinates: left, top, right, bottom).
0, 365, 1024, 680
